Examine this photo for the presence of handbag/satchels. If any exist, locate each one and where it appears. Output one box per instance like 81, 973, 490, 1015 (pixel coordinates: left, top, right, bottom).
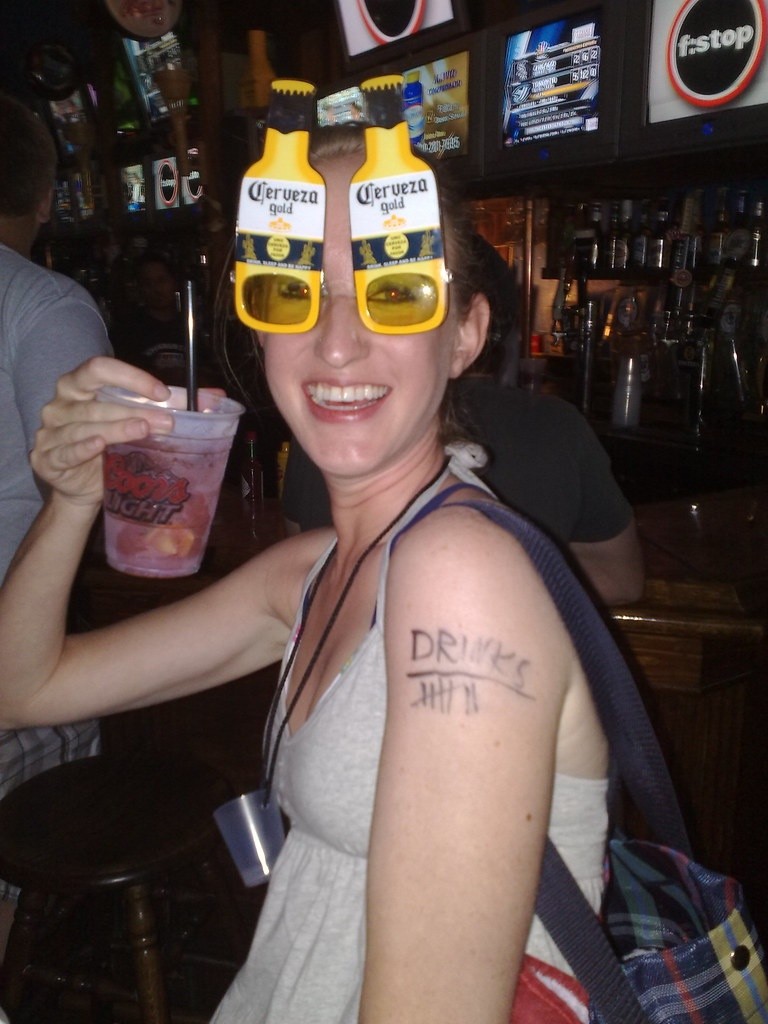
435, 499, 768, 1024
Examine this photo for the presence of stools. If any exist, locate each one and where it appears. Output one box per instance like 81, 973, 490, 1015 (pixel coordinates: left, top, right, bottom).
0, 753, 235, 1024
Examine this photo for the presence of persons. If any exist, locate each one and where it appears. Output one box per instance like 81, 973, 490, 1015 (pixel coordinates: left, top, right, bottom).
0, 94, 116, 969
111, 250, 207, 387
280, 230, 646, 605
0, 74, 609, 1024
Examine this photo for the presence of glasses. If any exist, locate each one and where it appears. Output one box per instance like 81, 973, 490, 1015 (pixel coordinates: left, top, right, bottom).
229, 74, 481, 335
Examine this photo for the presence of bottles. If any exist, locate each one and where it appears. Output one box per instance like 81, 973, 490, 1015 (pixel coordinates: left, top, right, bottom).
588, 201, 601, 270
400, 73, 425, 144
707, 185, 731, 258
730, 190, 752, 265
635, 198, 651, 274
615, 199, 636, 275
607, 200, 621, 273
748, 194, 766, 269
242, 434, 263, 522
677, 304, 699, 380
648, 196, 673, 273
654, 311, 678, 379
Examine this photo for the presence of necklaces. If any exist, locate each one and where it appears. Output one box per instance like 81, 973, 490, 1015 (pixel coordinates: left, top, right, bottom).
214, 451, 452, 889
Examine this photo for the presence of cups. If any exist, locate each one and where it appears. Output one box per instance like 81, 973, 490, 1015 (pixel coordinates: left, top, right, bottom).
213, 789, 286, 889
611, 354, 641, 426
95, 384, 245, 579
521, 358, 547, 395
